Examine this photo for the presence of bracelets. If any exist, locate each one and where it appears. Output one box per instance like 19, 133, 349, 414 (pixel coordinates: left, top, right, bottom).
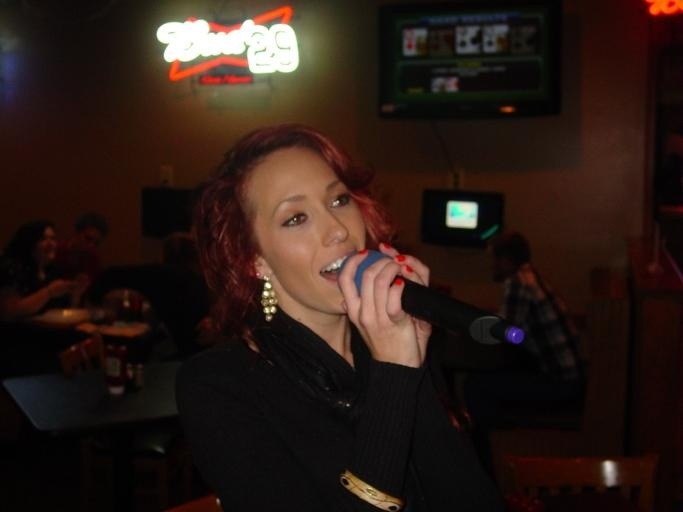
336, 469, 403, 511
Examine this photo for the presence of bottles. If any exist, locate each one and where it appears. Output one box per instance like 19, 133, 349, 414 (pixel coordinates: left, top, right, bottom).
125, 363, 145, 391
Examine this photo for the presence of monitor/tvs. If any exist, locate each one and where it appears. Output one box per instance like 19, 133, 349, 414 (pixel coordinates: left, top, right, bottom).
418, 187, 505, 249
375, 3, 563, 120
139, 187, 196, 238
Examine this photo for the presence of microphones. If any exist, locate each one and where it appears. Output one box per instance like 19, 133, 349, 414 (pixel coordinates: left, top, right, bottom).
336, 248, 526, 346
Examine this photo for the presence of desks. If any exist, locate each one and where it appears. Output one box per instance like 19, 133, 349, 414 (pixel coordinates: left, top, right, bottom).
2, 360, 178, 510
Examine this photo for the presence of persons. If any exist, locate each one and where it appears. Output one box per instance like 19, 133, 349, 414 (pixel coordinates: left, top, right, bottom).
463, 228, 586, 480
173, 122, 515, 511
0, 219, 92, 379
59, 212, 110, 339
